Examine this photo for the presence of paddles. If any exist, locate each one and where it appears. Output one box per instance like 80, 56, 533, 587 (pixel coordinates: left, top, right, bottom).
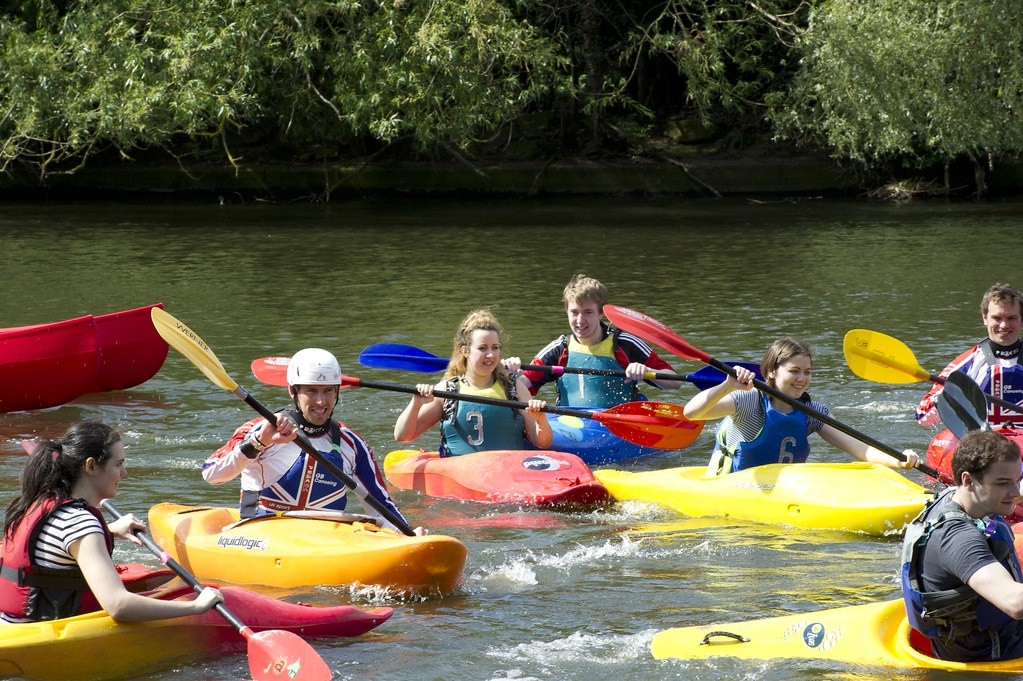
359, 342, 766, 391
149, 303, 420, 538
843, 327, 1023, 414
599, 298, 955, 489
19, 434, 333, 681
248, 354, 707, 449
933, 370, 991, 440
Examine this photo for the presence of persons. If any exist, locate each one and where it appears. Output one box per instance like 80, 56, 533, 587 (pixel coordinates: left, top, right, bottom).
202, 347, 430, 537
683, 336, 924, 476
915, 282, 1023, 430
901, 430, 1023, 662
394, 310, 552, 459
501, 273, 682, 409
0, 419, 225, 623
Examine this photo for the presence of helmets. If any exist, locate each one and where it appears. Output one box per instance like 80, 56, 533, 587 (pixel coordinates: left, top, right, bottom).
287, 348, 341, 398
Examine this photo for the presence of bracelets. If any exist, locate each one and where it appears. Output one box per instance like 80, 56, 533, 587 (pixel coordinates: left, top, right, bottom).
250, 434, 266, 451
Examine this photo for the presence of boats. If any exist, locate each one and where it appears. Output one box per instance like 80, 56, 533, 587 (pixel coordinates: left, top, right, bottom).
384, 449, 609, 505
524, 408, 664, 464
147, 501, 467, 600
83, 303, 169, 394
925, 423, 1023, 526
0, 314, 106, 414
1, 564, 393, 681
650, 597, 1023, 673
592, 463, 938, 536
